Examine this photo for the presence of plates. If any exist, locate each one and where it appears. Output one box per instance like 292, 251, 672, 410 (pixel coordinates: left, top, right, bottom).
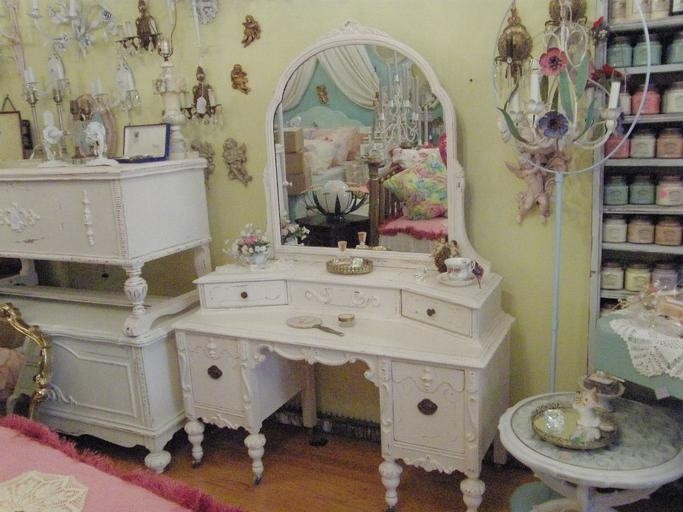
530, 400, 619, 450
438, 271, 479, 286
326, 258, 373, 275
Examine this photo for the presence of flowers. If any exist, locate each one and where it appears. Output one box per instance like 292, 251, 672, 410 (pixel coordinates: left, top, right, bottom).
222, 222, 271, 267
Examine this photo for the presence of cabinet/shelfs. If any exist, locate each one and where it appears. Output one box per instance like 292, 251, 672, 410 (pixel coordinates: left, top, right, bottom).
589, 1, 682, 376
1, 157, 214, 475
170, 260, 516, 512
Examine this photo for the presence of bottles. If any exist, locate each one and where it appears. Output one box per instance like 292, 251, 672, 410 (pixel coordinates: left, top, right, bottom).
333, 241, 353, 267
356, 231, 369, 250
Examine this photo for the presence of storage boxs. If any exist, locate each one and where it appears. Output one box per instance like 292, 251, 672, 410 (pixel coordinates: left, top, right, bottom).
274, 126, 311, 197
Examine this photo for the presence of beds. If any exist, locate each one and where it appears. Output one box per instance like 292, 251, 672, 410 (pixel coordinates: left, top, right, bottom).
276, 105, 368, 219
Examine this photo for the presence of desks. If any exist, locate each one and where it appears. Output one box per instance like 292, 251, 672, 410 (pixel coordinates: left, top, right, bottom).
293, 213, 370, 247
496, 392, 683, 512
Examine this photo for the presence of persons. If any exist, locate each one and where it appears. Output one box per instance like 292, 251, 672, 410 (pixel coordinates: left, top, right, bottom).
515, 152, 552, 225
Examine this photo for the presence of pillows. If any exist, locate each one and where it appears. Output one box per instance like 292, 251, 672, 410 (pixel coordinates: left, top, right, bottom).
383, 146, 449, 222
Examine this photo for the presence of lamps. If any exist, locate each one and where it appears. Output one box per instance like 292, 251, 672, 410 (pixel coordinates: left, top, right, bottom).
301, 179, 367, 224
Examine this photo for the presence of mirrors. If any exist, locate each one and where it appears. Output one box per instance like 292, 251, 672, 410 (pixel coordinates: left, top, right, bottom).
263, 17, 468, 261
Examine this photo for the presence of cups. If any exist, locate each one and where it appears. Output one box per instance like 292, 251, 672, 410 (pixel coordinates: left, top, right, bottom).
578, 374, 625, 412
444, 257, 475, 281
